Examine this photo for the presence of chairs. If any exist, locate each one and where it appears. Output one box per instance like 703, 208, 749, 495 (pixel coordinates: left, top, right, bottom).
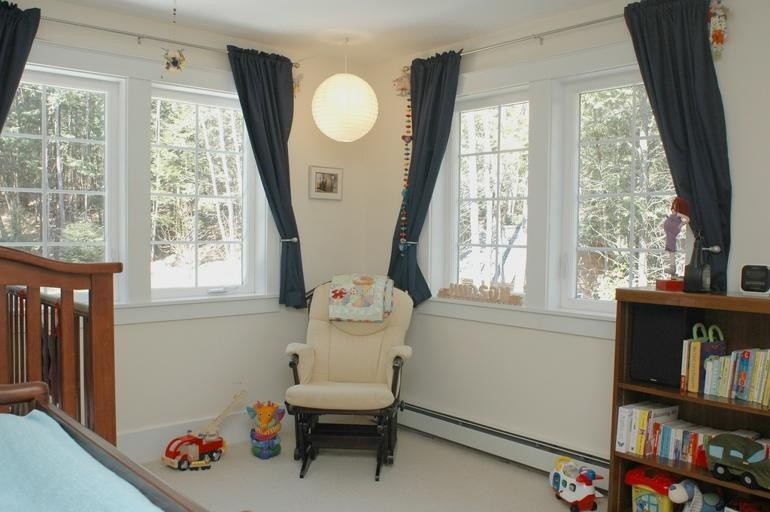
284, 275, 414, 482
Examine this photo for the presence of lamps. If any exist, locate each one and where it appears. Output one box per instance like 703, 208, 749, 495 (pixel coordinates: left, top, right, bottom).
311, 53, 381, 143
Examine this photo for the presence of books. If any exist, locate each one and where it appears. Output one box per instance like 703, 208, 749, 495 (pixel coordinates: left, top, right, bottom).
614, 346, 769, 469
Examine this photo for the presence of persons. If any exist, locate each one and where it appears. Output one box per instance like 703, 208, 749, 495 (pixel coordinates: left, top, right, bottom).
653, 196, 691, 276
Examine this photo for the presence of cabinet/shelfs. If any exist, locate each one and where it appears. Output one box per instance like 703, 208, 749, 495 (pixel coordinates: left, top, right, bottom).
608, 286, 770, 512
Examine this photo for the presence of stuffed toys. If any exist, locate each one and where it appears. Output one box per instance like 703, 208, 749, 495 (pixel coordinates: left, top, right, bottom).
667, 478, 739, 512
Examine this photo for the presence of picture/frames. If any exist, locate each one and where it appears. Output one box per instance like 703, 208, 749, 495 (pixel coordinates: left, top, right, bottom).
309, 167, 342, 201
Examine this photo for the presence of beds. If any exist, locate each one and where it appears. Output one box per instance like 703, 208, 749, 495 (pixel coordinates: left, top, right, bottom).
0, 381, 210, 512
